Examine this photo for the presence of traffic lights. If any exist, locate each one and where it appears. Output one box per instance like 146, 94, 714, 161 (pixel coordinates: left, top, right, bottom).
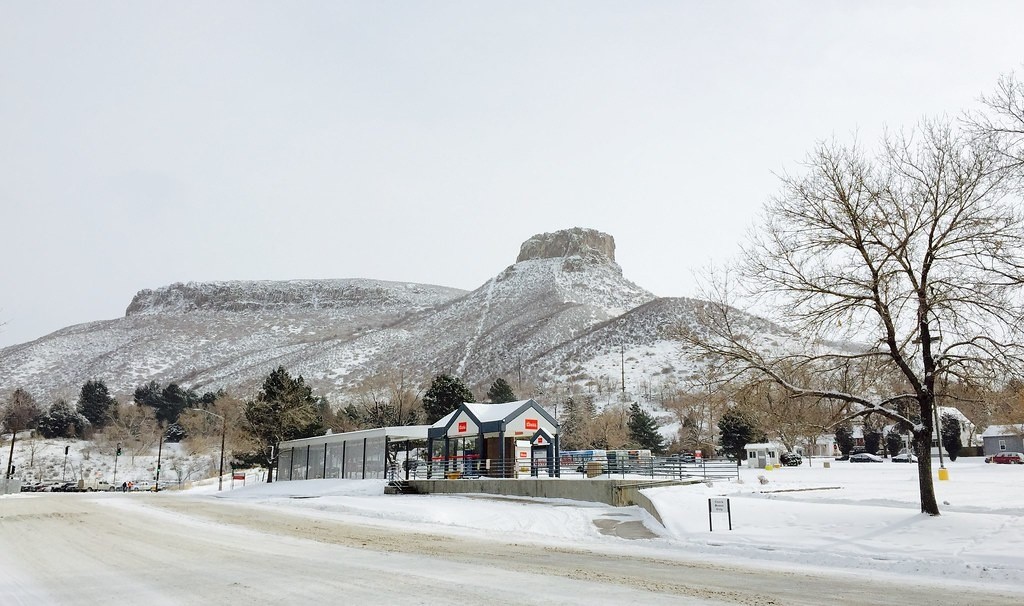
117, 447, 122, 456
11, 466, 15, 474
157, 464, 161, 472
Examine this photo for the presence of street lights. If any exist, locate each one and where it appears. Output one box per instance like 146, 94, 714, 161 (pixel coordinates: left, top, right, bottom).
553, 403, 557, 420
193, 409, 226, 491
912, 336, 949, 480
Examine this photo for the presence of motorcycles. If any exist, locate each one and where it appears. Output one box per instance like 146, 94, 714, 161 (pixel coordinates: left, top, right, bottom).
123, 487, 127, 492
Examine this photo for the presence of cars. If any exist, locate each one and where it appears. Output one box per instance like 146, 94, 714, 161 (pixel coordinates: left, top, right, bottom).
984, 452, 1024, 464
835, 454, 849, 461
850, 453, 883, 463
401, 460, 418, 471
679, 453, 693, 461
892, 453, 918, 463
576, 460, 614, 475
21, 479, 196, 492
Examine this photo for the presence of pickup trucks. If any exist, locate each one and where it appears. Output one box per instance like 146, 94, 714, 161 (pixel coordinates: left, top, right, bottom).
666, 454, 679, 462
